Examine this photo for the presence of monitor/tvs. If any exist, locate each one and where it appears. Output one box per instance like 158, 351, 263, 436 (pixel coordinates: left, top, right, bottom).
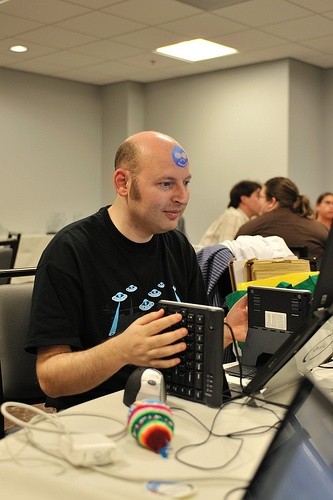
238, 375, 333, 500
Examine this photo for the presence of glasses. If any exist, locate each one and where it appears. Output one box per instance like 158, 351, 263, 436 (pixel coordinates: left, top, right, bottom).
257, 195, 268, 201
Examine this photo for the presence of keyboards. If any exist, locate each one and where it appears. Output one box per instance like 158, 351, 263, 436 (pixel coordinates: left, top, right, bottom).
157, 300, 225, 408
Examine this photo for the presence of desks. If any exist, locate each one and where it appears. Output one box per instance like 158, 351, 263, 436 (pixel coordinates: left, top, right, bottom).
0, 361, 305, 500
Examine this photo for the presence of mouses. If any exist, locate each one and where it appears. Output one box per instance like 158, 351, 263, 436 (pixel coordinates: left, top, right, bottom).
123, 367, 166, 407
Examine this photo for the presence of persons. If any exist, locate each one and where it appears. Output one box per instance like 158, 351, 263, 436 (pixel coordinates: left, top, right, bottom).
311, 192, 333, 231
233, 176, 329, 272
197, 180, 263, 248
22, 131, 249, 414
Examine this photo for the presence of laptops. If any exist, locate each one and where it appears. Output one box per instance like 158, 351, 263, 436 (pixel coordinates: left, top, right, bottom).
243, 217, 333, 396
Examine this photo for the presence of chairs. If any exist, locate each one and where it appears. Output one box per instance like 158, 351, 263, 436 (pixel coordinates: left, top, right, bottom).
0, 266, 60, 440
0, 232, 21, 285
218, 245, 309, 317
